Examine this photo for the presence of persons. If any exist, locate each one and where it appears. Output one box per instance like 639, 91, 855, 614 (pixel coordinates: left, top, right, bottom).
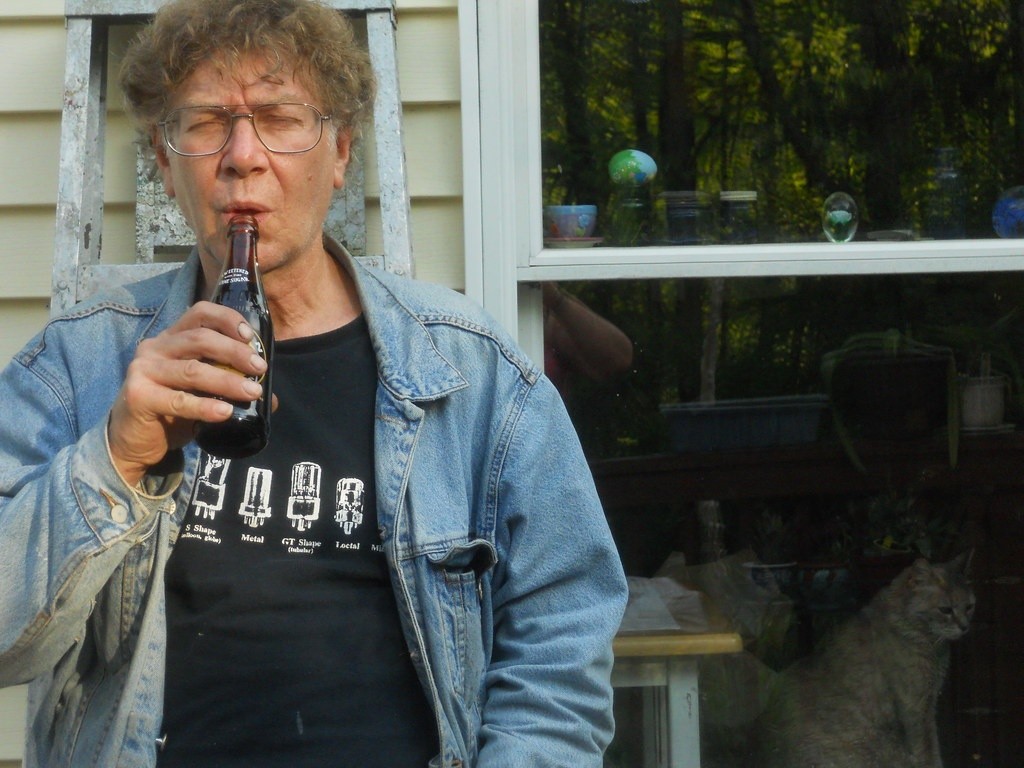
544, 280, 632, 417
1, 1, 629, 767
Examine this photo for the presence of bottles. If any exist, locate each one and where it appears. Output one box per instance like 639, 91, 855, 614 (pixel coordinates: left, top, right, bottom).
190, 214, 273, 459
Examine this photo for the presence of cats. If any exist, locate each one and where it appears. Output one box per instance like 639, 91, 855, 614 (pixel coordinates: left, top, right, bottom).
760, 548, 976, 768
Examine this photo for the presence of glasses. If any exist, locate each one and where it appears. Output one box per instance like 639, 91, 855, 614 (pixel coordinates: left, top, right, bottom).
157, 101, 333, 157
